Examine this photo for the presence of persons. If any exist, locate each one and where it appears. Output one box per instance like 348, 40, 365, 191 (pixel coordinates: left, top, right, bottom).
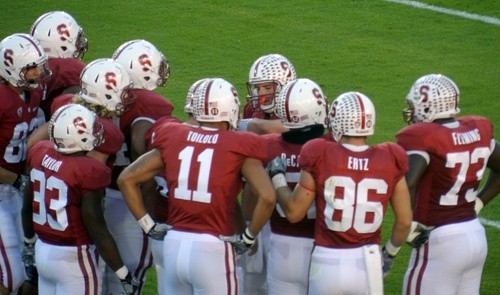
266, 92, 413, 295
238, 54, 296, 133
21, 102, 140, 295
118, 77, 278, 294
48, 58, 130, 164
0, 32, 53, 295
104, 39, 174, 295
141, 78, 211, 295
31, 11, 88, 99
395, 74, 500, 295
241, 78, 329, 295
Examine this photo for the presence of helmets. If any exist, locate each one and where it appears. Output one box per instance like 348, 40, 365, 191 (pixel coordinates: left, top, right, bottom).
78, 57, 133, 116
112, 39, 168, 92
328, 91, 376, 143
404, 73, 462, 123
30, 11, 85, 60
247, 53, 296, 114
0, 33, 48, 90
185, 78, 240, 130
48, 103, 104, 155
275, 77, 328, 129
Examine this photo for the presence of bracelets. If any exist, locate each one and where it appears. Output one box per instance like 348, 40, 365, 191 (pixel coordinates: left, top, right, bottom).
242, 228, 255, 244
271, 173, 287, 190
138, 213, 155, 234
116, 266, 129, 279
475, 197, 483, 216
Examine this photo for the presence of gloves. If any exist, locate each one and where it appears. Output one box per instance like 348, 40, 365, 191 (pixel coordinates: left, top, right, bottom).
266, 156, 286, 190
115, 264, 143, 295
137, 214, 173, 241
219, 226, 255, 258
24, 240, 37, 283
382, 240, 401, 278
